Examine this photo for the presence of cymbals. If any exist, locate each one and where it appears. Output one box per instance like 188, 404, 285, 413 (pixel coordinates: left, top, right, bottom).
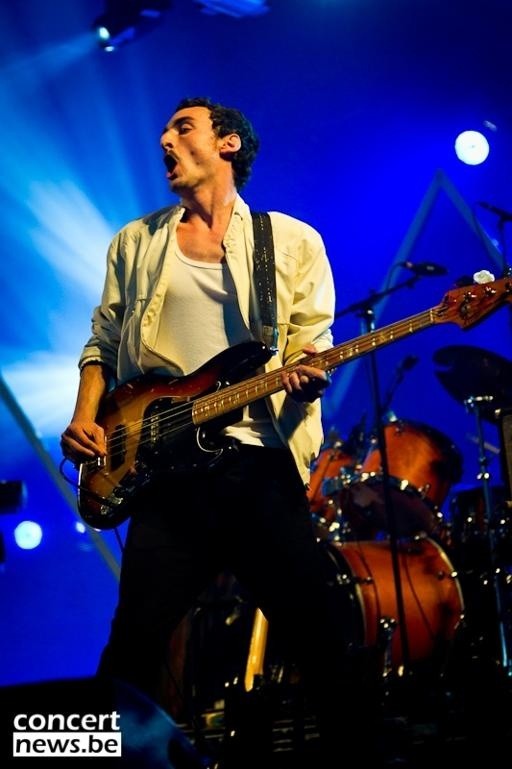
435, 345, 511, 422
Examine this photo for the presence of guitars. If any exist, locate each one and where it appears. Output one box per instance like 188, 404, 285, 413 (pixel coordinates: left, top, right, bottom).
311, 531, 468, 672
76, 267, 511, 531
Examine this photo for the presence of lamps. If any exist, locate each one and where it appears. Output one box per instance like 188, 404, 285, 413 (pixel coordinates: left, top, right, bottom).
94, 0, 159, 43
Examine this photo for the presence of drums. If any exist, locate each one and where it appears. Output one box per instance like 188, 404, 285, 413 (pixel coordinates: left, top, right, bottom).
305, 443, 358, 540
339, 419, 465, 538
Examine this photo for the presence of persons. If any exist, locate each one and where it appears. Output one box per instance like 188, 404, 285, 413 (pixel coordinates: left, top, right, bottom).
60, 96, 396, 767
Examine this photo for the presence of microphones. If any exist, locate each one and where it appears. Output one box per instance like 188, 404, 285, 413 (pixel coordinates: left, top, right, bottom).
401, 261, 448, 276
481, 202, 512, 221
